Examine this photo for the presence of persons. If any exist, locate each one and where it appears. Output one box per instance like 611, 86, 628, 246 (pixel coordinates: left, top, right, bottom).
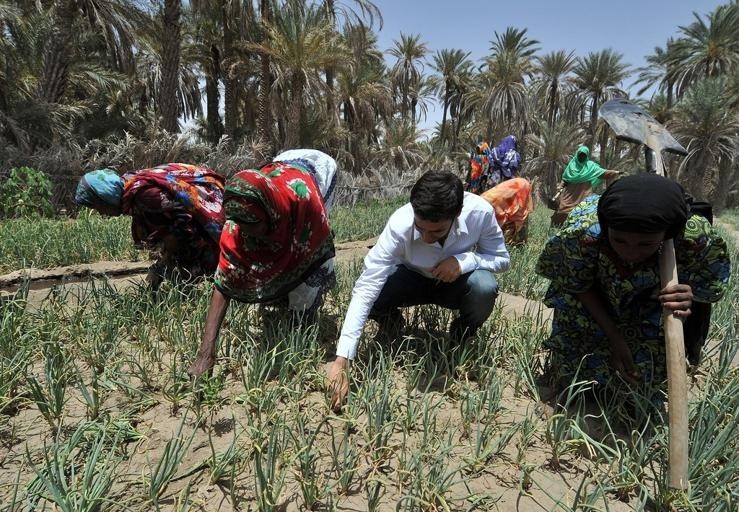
481, 178, 532, 247
534, 172, 730, 405
187, 148, 338, 387
327, 169, 510, 414
75, 164, 228, 283
486, 135, 522, 189
551, 147, 623, 215
465, 143, 490, 192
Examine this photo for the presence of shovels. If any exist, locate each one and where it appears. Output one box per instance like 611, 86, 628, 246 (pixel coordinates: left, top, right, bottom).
599, 97, 689, 490
